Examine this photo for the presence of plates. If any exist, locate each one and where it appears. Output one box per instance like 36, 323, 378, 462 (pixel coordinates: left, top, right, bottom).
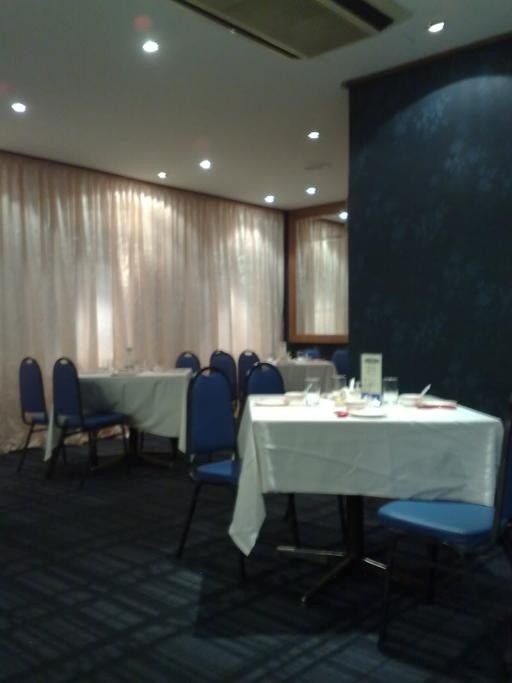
350, 410, 386, 417
257, 398, 286, 405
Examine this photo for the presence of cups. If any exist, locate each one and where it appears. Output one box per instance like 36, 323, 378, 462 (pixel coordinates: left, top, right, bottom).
285, 390, 306, 405
304, 377, 322, 405
329, 375, 347, 402
382, 377, 399, 403
400, 393, 421, 405
344, 397, 368, 412
